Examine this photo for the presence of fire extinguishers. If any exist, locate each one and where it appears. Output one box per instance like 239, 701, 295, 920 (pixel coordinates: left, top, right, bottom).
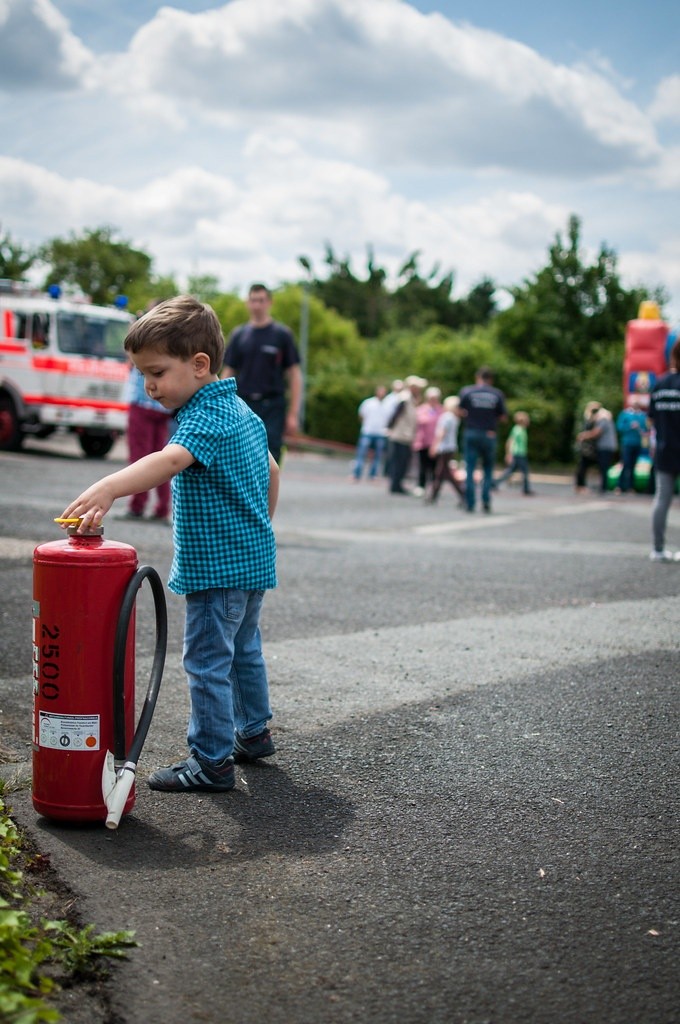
29, 515, 169, 832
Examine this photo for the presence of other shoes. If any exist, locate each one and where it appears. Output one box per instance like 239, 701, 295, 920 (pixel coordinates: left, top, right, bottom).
114, 512, 143, 522
144, 516, 172, 526
650, 549, 680, 562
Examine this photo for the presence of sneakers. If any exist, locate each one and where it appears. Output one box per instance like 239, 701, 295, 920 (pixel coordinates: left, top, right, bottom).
148, 747, 235, 792
231, 727, 276, 763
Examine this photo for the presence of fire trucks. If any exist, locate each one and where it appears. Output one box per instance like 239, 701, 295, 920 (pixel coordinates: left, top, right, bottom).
0, 275, 145, 459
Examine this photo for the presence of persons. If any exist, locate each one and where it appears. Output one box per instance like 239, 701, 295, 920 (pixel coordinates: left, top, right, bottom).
58, 296, 280, 793
222, 284, 303, 467
352, 365, 533, 514
575, 401, 656, 495
649, 340, 680, 561
116, 299, 172, 521
